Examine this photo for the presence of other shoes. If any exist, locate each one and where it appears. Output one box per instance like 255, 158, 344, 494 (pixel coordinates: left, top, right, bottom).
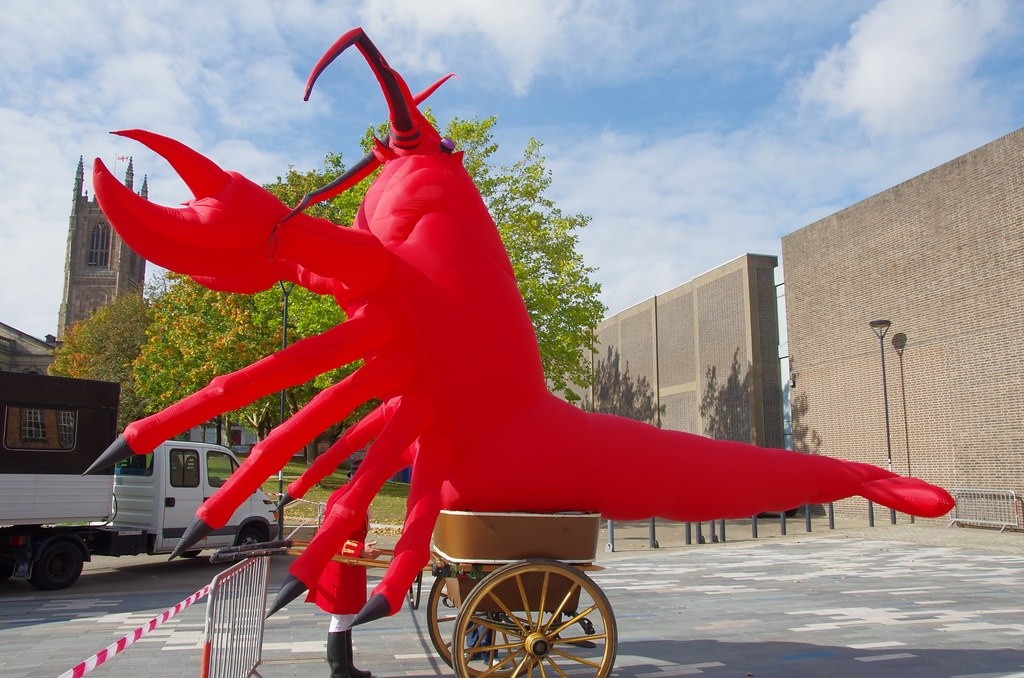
463, 652, 481, 661
492, 657, 509, 669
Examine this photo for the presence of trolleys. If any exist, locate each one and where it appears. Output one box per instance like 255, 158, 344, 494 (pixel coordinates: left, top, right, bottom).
210, 509, 618, 678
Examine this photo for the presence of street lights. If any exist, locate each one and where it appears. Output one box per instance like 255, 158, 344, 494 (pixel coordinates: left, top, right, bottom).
869, 320, 897, 524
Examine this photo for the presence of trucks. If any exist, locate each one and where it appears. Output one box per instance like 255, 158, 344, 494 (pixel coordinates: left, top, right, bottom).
0, 439, 281, 591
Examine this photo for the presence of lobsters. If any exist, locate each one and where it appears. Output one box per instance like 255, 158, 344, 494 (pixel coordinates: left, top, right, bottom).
86, 25, 957, 628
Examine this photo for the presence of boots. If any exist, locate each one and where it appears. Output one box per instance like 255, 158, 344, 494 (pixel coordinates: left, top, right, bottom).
327, 628, 371, 678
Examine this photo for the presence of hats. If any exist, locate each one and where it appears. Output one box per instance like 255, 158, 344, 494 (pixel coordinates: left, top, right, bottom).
346, 458, 367, 480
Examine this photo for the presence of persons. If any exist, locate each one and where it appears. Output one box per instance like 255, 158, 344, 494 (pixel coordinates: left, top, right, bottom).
302, 458, 389, 678
465, 611, 506, 670
